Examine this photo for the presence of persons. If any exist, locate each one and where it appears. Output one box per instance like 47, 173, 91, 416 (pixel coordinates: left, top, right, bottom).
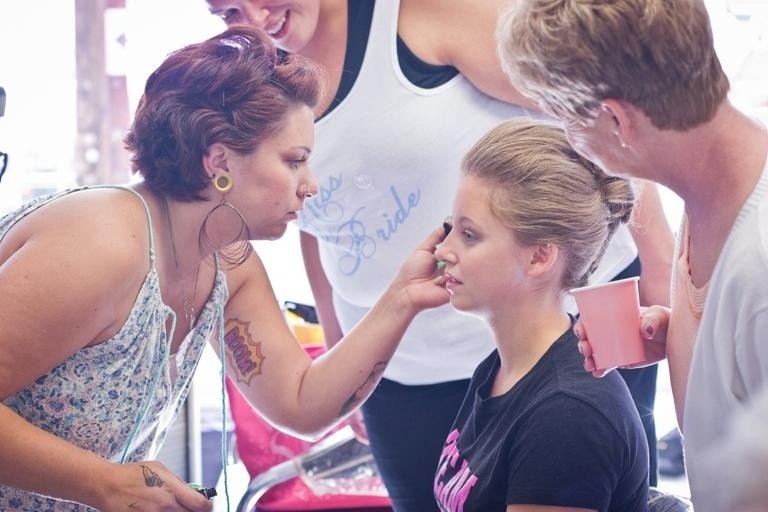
0, 25, 452, 511
425, 113, 654, 511
498, 0, 768, 510
201, 1, 677, 511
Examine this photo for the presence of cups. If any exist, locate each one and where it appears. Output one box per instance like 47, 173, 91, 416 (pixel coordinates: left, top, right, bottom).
566, 272, 648, 374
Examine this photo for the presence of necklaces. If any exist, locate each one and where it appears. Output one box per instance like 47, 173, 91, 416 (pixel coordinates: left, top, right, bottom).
164, 203, 201, 335
